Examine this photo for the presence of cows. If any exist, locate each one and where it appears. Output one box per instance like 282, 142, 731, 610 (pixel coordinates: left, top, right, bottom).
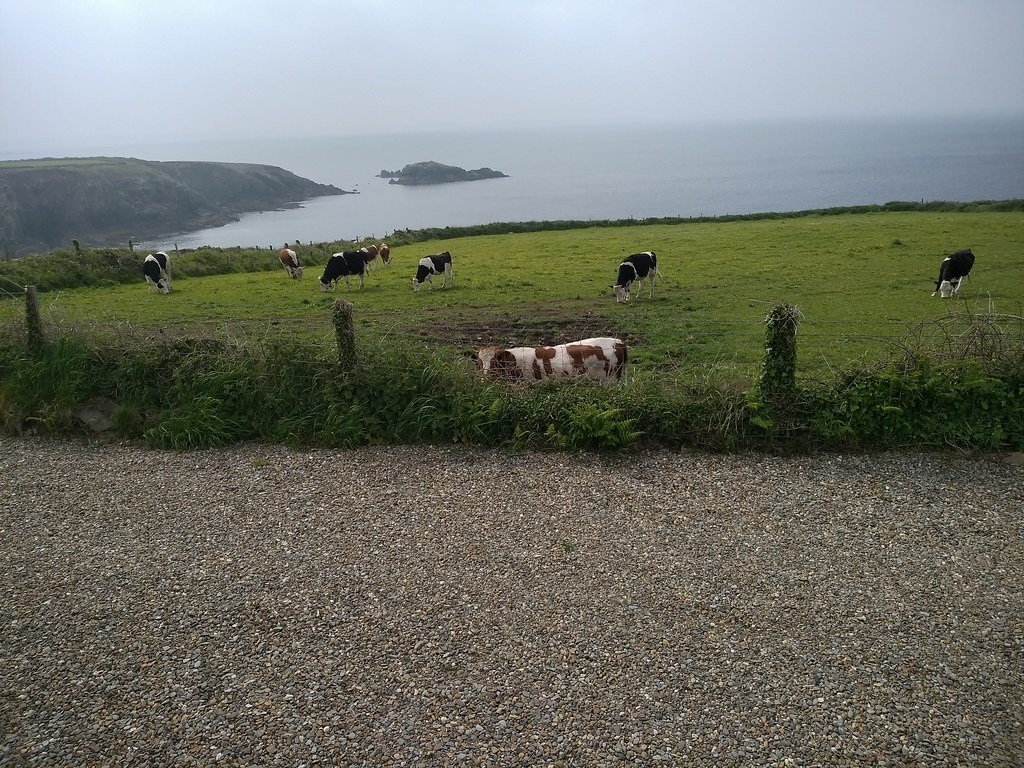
318, 242, 391, 293
931, 248, 975, 298
609, 251, 657, 303
411, 251, 454, 292
279, 249, 305, 281
143, 251, 172, 294
475, 337, 628, 382
